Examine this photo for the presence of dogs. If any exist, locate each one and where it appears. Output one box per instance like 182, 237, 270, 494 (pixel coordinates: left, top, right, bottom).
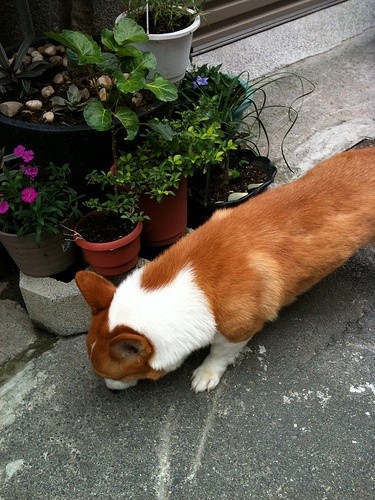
75, 146, 375, 394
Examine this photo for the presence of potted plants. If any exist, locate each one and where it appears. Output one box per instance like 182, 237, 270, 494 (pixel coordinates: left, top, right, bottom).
114, 0, 211, 82
0, 35, 178, 202
45, 17, 179, 277
172, 70, 319, 223
86, 93, 240, 248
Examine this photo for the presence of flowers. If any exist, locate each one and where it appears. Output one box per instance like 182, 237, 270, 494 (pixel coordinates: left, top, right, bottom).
175, 64, 240, 104
0, 142, 87, 244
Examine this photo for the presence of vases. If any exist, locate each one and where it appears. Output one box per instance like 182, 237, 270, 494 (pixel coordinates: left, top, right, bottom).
0, 215, 73, 280
180, 76, 255, 137
